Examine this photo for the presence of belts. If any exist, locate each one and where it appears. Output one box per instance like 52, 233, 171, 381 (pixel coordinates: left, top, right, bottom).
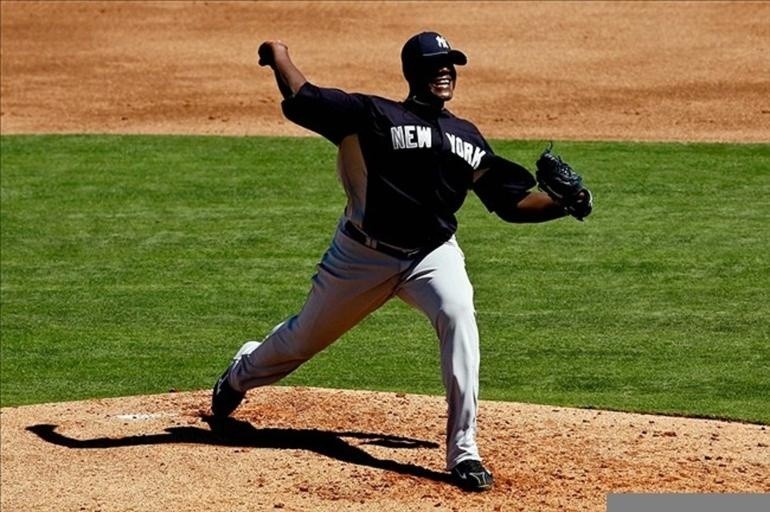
342, 206, 421, 261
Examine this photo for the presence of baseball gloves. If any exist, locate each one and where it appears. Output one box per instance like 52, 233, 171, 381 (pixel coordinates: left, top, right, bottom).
535, 149, 593, 222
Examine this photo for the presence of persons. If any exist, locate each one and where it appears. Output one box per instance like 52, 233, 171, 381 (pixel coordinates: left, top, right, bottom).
211, 32, 593, 486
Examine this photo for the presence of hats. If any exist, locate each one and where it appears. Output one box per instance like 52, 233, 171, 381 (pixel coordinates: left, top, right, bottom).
401, 32, 467, 67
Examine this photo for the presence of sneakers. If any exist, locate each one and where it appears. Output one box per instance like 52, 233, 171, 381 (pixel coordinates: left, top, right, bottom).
451, 460, 494, 490
212, 360, 245, 420
